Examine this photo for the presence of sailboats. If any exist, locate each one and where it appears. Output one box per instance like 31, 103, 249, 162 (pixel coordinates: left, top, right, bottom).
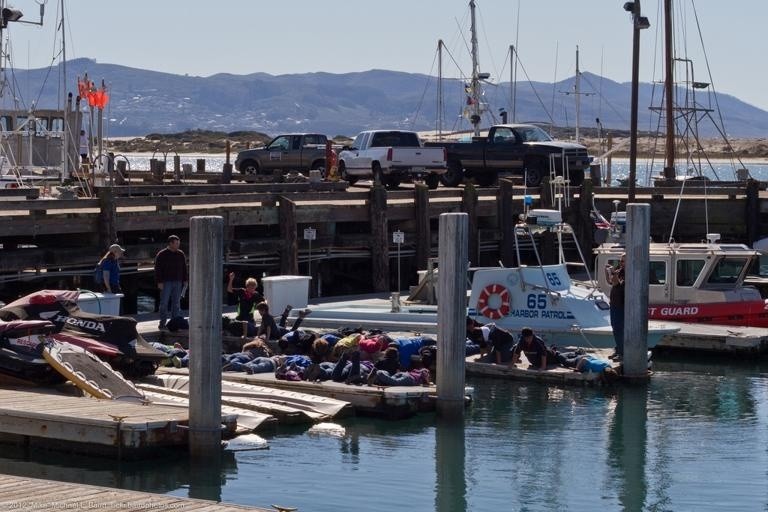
644, 0, 742, 188
409, 0, 639, 157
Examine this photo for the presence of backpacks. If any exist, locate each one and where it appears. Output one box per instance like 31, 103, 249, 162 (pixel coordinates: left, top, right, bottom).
95, 262, 103, 284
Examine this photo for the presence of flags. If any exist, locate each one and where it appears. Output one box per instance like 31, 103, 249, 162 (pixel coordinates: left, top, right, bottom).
593, 219, 610, 230
79, 80, 109, 109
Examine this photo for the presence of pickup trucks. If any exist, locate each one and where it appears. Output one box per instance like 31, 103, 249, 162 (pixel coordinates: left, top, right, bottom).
424, 123, 593, 186
234, 133, 345, 183
338, 129, 449, 188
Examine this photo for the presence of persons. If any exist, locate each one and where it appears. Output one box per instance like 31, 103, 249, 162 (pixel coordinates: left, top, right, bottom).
572, 348, 617, 380
604, 251, 625, 362
504, 327, 590, 373
154, 235, 188, 329
93, 244, 126, 294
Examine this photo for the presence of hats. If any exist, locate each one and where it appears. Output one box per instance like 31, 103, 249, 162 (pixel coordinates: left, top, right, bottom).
159, 354, 181, 369
109, 244, 125, 254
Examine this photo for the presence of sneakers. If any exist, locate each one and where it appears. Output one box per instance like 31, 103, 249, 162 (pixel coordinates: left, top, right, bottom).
286, 305, 294, 311
299, 309, 312, 318
219, 360, 254, 375
343, 335, 361, 349
